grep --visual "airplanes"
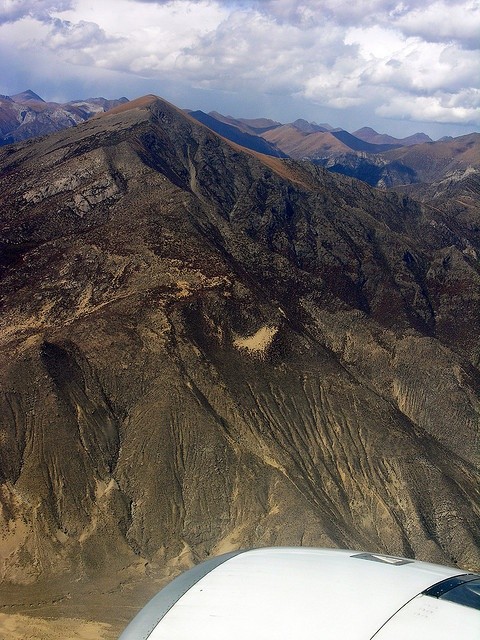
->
[120,545,480,640]
